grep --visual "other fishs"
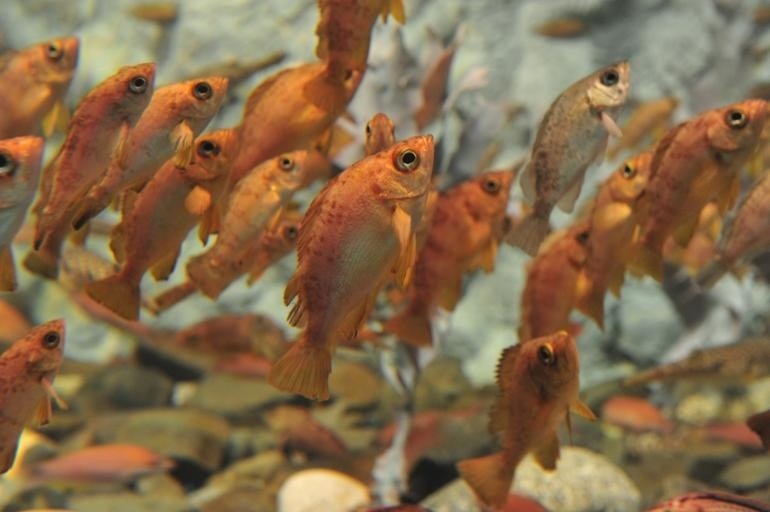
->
[0,0,769,512]
